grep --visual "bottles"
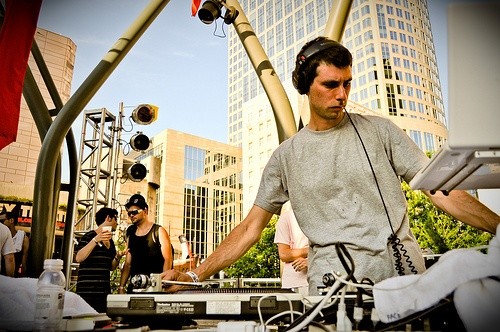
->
[32,258,66,332]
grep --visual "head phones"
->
[291,39,341,90]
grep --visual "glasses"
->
[0,218,11,224]
[127,209,144,217]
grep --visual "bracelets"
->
[92,238,102,247]
[186,271,199,282]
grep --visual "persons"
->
[161,36,500,332]
[75,207,130,314]
[3,211,29,278]
[118,194,174,294]
[0,222,15,277]
[273,200,309,293]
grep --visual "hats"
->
[123,194,149,210]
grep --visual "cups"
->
[102,226,112,240]
